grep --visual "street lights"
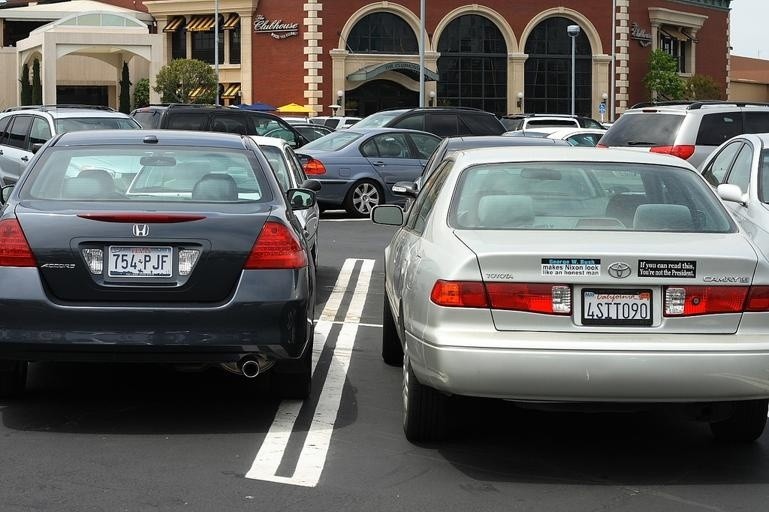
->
[566,24,581,114]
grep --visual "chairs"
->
[278,172,285,180]
[268,159,282,171]
[633,203,697,232]
[363,144,384,157]
[75,169,115,196]
[174,161,211,191]
[604,189,648,220]
[42,126,50,139]
[213,123,226,131]
[378,143,402,156]
[230,125,246,133]
[62,178,102,199]
[477,193,535,231]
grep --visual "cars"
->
[369,140,769,446]
[254,102,616,148]
[0,127,318,402]
[291,126,444,220]
[697,132,768,263]
[0,101,186,211]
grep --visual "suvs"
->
[128,101,313,150]
[592,99,769,194]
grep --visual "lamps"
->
[337,90,343,106]
[601,93,608,105]
[517,92,523,109]
[429,91,435,107]
